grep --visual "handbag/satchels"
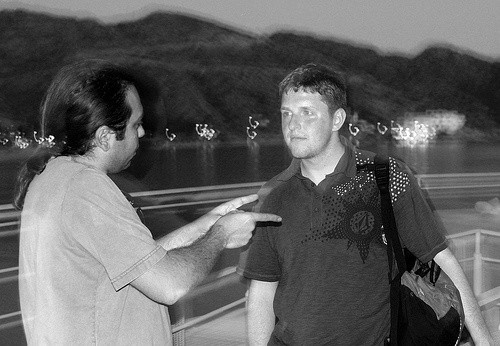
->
[374,152,465,346]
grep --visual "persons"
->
[236,63,495,346]
[11,61,284,346]
